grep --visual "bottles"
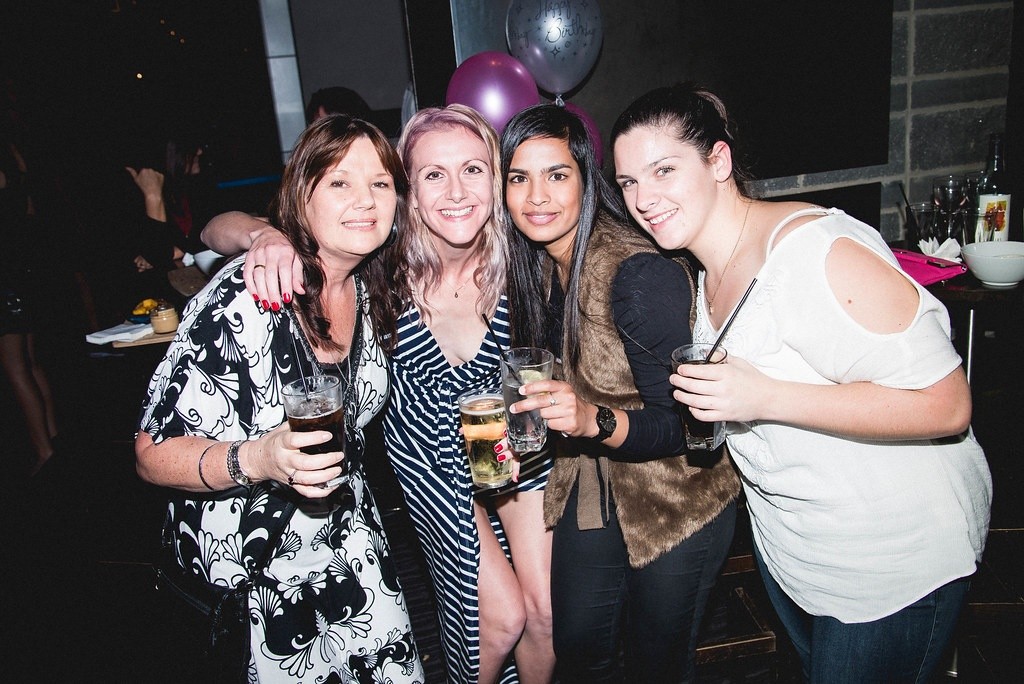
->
[974,131,1012,243]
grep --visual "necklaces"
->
[331,352,355,400]
[443,278,470,298]
[705,199,752,315]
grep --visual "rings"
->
[288,470,297,484]
[254,265,265,269]
[550,398,556,405]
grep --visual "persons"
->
[498,105,742,684]
[306,87,370,124]
[125,114,202,294]
[609,85,993,684]
[136,115,426,684]
[200,102,554,684]
[0,139,60,476]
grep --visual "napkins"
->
[917,237,962,263]
[86,322,154,345]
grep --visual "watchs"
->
[592,405,617,442]
[232,439,255,486]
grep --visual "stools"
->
[695,528,1024,684]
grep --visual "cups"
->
[905,204,938,254]
[500,347,553,452]
[457,387,513,487]
[962,212,997,245]
[282,375,348,487]
[670,343,727,451]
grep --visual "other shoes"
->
[24,453,65,481]
[51,434,64,448]
[90,344,128,358]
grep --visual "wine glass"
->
[933,175,968,239]
[963,175,980,228]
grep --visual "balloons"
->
[565,102,603,170]
[446,51,539,135]
[506,0,603,95]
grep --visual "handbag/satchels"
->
[137,565,251,684]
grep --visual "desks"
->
[111,320,174,347]
[888,240,1024,385]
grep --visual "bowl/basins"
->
[960,240,1024,287]
[193,250,226,277]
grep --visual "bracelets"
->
[199,444,215,490]
[227,440,241,481]
